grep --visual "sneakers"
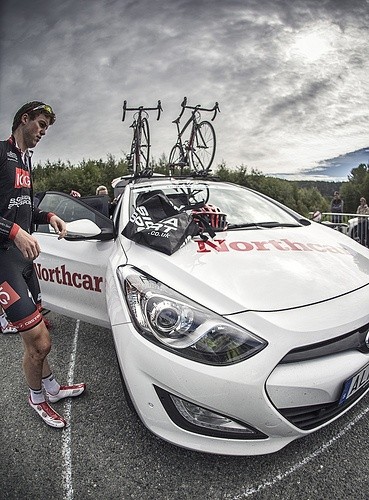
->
[1,323,19,333]
[28,395,66,428]
[46,383,86,403]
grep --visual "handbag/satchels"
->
[119,194,215,256]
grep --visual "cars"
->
[33,173,369,457]
[346,216,369,242]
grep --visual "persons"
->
[0,100,85,428]
[68,185,119,218]
[331,191,343,223]
[357,197,369,247]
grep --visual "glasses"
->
[26,105,52,114]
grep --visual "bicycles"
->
[120,97,164,176]
[169,97,220,174]
